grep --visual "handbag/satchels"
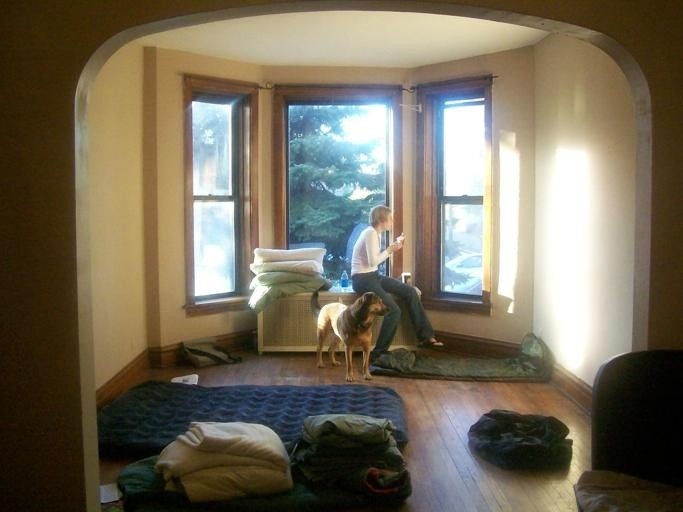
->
[180,341,233,368]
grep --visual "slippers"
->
[417,340,447,350]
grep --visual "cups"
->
[397,237,405,245]
[400,272,410,285]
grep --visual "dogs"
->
[310,290,392,382]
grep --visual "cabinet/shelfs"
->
[257,289,421,354]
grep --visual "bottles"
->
[339,270,348,290]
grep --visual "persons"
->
[350,205,447,363]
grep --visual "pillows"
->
[248,247,326,309]
